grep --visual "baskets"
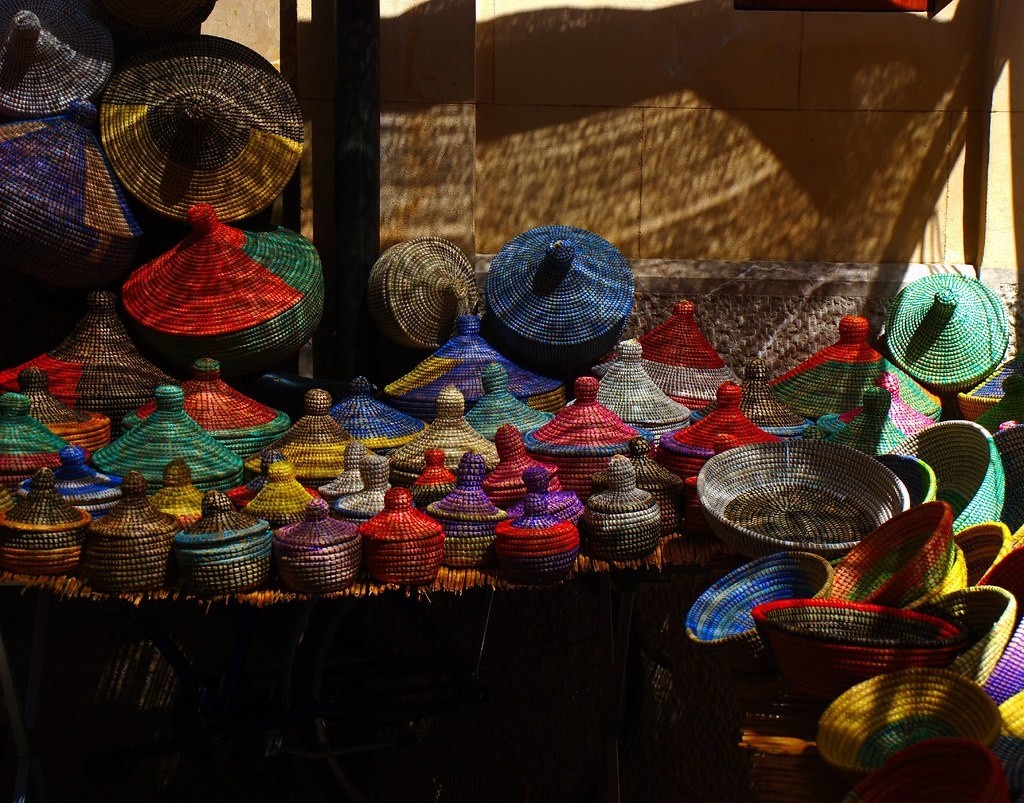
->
[681,418,1024,803]
